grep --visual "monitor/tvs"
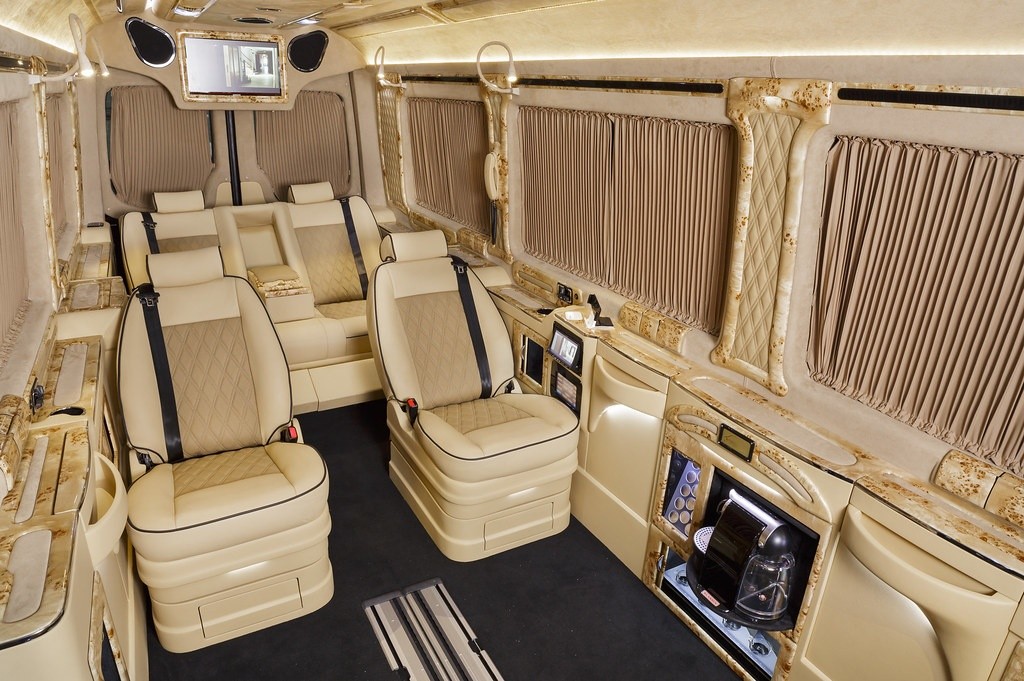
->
[555,372,577,408]
[548,328,581,369]
[101,621,121,681]
[176,29,289,105]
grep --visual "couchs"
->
[116,246,335,653]
[366,230,581,563]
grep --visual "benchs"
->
[118,180,385,416]
